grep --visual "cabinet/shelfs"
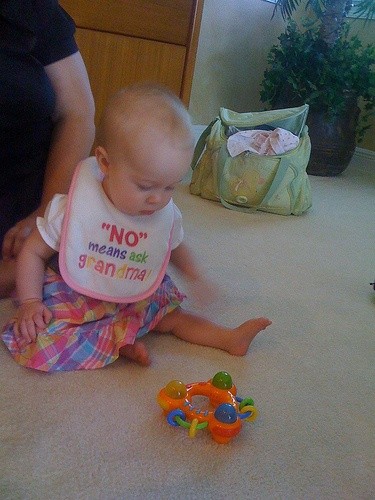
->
[58,0,205,158]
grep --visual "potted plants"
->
[257,0,375,177]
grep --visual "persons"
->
[0,85,273,372]
[0,0,96,298]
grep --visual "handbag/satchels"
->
[189,104,313,216]
